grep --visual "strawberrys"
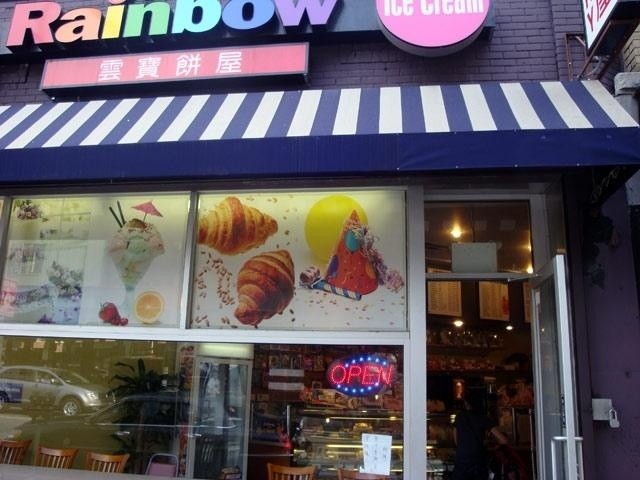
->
[98,302,119,322]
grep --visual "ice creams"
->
[104,200,165,292]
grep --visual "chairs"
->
[338,468,391,479]
[142,453,180,475]
[267,462,317,478]
[1,439,30,466]
[85,451,130,472]
[32,445,78,468]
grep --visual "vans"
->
[1,364,118,418]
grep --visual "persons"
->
[453,388,529,480]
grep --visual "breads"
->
[196,196,278,255]
[234,250,295,326]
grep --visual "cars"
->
[11,391,244,479]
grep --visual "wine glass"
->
[108,251,156,323]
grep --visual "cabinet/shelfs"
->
[291,407,446,480]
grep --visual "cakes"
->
[300,264,319,284]
[0,200,60,322]
[323,210,379,295]
